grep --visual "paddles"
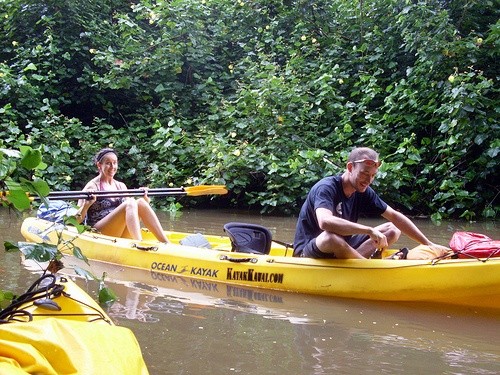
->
[0,185,228,203]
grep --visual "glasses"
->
[350,159,383,168]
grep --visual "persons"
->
[292,147,435,259]
[75,148,169,243]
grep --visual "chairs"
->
[223,222,295,255]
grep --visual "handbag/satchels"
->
[37,199,78,224]
[450,231,500,259]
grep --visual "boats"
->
[0,265,150,374]
[21,217,500,310]
